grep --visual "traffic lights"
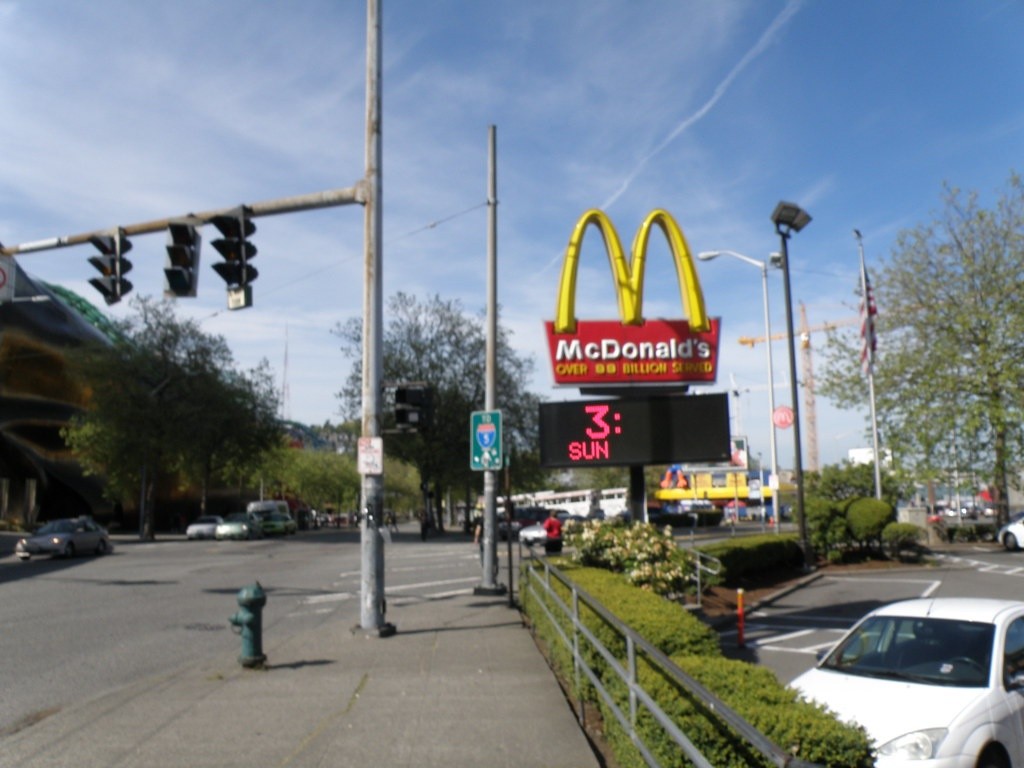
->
[209,203,259,288]
[87,235,133,303]
[165,222,202,298]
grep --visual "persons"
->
[474,514,498,581]
[386,512,398,535]
[421,512,428,541]
[544,512,562,555]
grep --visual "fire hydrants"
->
[228,579,267,669]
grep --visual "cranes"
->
[738,298,858,475]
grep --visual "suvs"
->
[214,511,263,541]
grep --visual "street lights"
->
[697,249,779,535]
[769,199,816,574]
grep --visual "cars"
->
[997,515,1024,550]
[260,511,298,537]
[783,596,1024,768]
[918,491,1003,544]
[296,507,395,531]
[664,499,724,528]
[467,504,587,547]
[14,517,112,561]
[186,515,223,541]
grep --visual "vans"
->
[244,499,291,524]
